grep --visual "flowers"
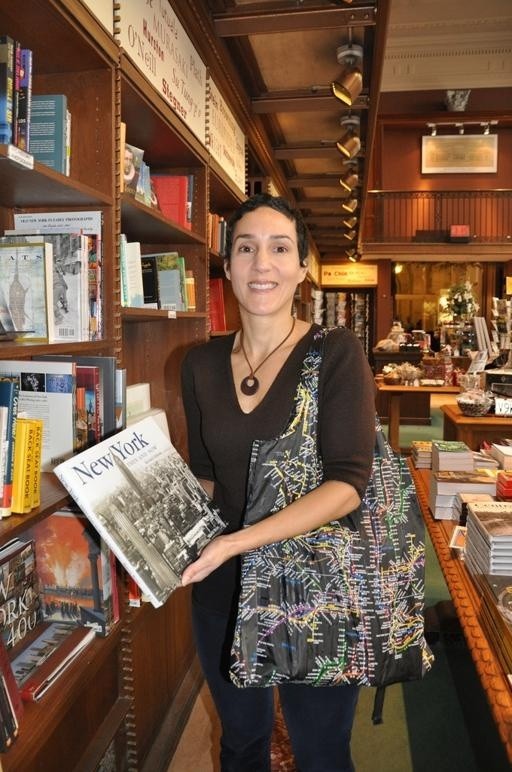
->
[443,280,480,315]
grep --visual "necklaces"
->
[237,313,297,398]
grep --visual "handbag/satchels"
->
[223,325,438,690]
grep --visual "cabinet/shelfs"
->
[0,1,250,772]
[491,295,512,370]
[290,260,378,376]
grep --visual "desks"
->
[406,455,512,772]
[373,374,463,451]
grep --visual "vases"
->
[451,315,463,324]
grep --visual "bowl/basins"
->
[455,397,491,417]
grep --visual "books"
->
[3,356,126,473]
[121,121,228,331]
[0,653,25,747]
[1,209,104,345]
[0,38,72,177]
[20,505,142,638]
[126,383,169,439]
[410,438,512,685]
[2,539,43,648]
[3,380,42,518]
[5,622,96,701]
[53,414,224,609]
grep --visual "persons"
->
[182,193,375,771]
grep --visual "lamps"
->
[341,192,358,241]
[344,247,359,263]
[338,158,359,192]
[336,115,362,159]
[331,45,365,106]
